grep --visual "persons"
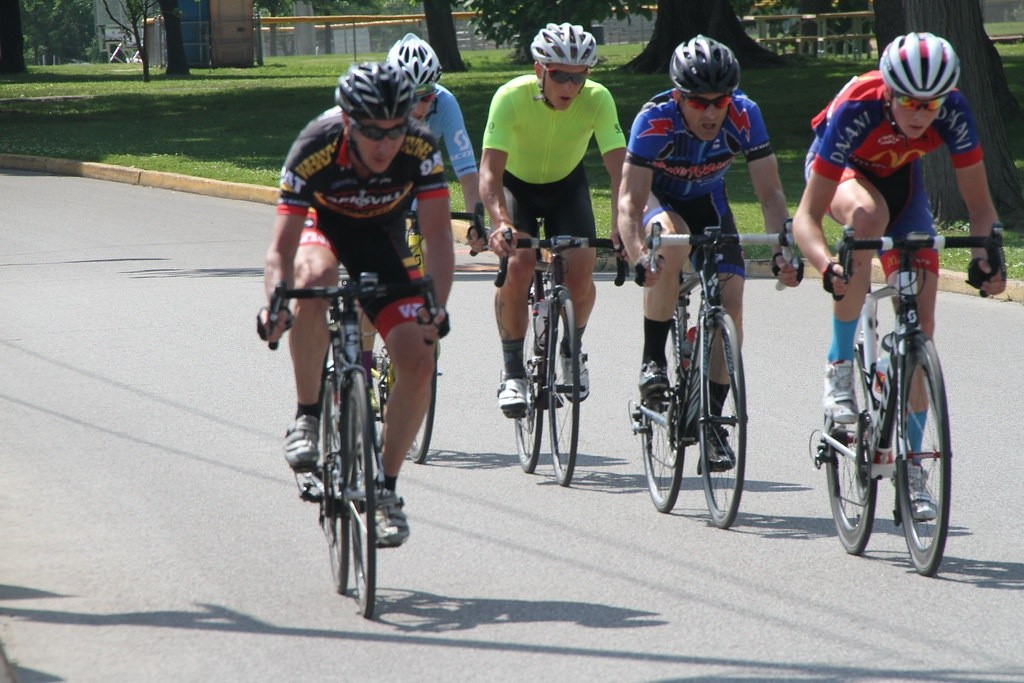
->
[356,32,491,414]
[477,23,632,410]
[792,32,1006,520]
[617,33,804,471]
[258,60,455,544]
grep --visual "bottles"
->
[680,326,695,368]
[536,297,550,338]
[872,334,895,401]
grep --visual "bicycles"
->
[494,222,631,488]
[808,223,1007,576]
[628,217,804,529]
[264,278,434,620]
[363,201,487,467]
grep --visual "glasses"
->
[542,64,591,85]
[892,88,948,110]
[349,116,409,141]
[681,91,733,110]
[413,88,440,105]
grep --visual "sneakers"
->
[640,360,669,393]
[697,431,736,473]
[559,342,589,403]
[821,346,859,423]
[282,414,320,466]
[907,461,937,520]
[497,368,528,411]
[373,470,410,545]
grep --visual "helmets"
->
[879,32,961,97]
[669,34,741,94]
[382,33,442,95]
[335,61,416,120]
[530,22,598,67]
